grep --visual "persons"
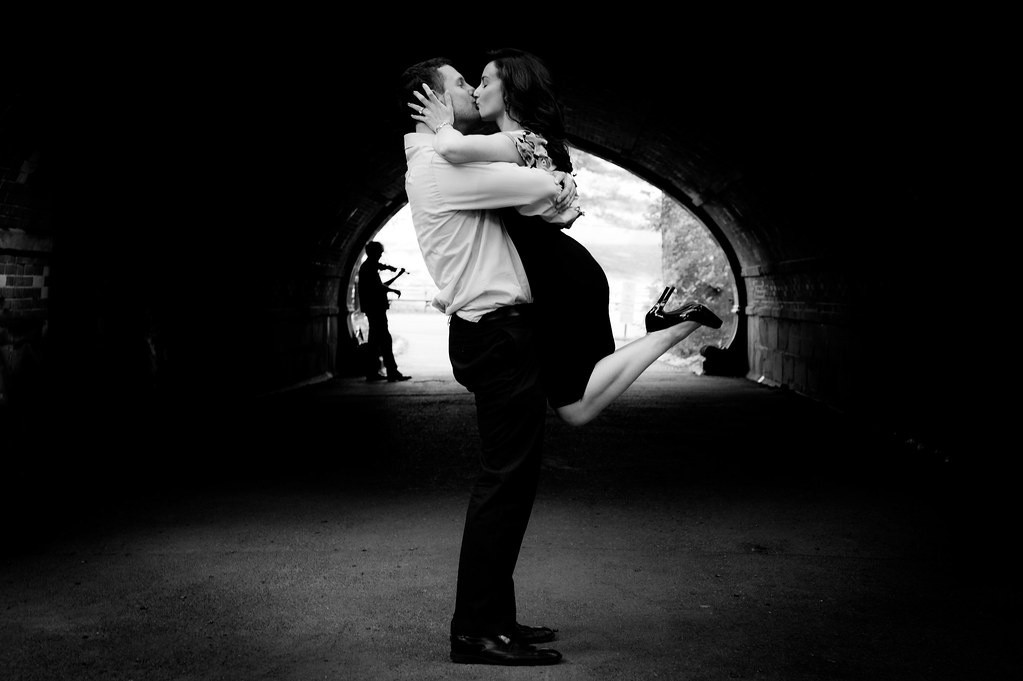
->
[358,241,412,384]
[408,47,723,427]
[403,56,584,667]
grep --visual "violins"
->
[378,262,410,274]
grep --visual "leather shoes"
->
[451,634,563,665]
[502,621,555,644]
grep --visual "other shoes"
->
[387,370,412,382]
[366,375,386,382]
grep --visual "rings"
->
[419,107,425,115]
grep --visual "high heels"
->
[645,285,724,331]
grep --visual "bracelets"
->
[435,121,454,134]
